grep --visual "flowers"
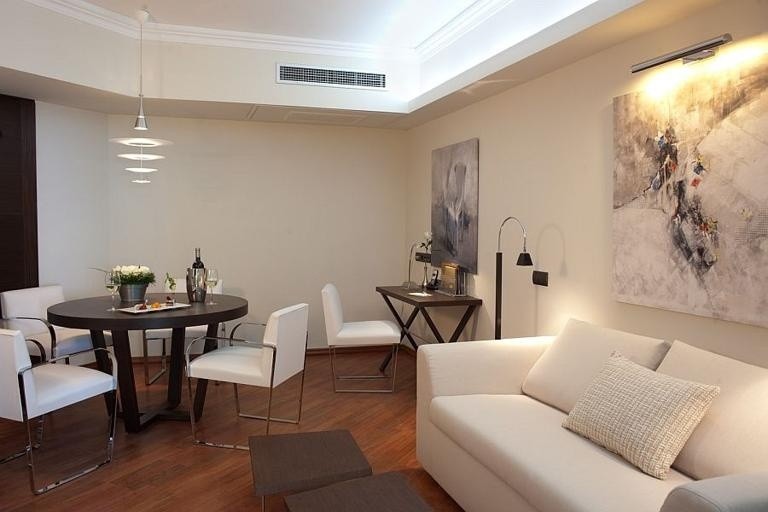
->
[101,263,157,285]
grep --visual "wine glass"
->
[105,272,122,311]
[204,268,219,305]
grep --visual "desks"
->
[372,284,483,376]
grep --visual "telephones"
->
[426,269,442,291]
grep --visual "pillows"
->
[562,352,720,478]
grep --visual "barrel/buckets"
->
[115,283,147,302]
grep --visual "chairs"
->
[136,277,229,384]
[2,326,121,496]
[1,282,117,379]
[180,302,314,452]
[321,284,400,394]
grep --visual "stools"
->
[238,424,372,512]
[277,466,432,512]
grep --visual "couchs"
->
[406,321,767,511]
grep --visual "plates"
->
[116,302,191,314]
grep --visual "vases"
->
[112,282,142,307]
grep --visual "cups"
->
[163,278,176,305]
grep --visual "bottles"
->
[186,247,207,302]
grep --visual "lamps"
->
[493,214,534,342]
[131,4,149,132]
[624,31,733,76]
